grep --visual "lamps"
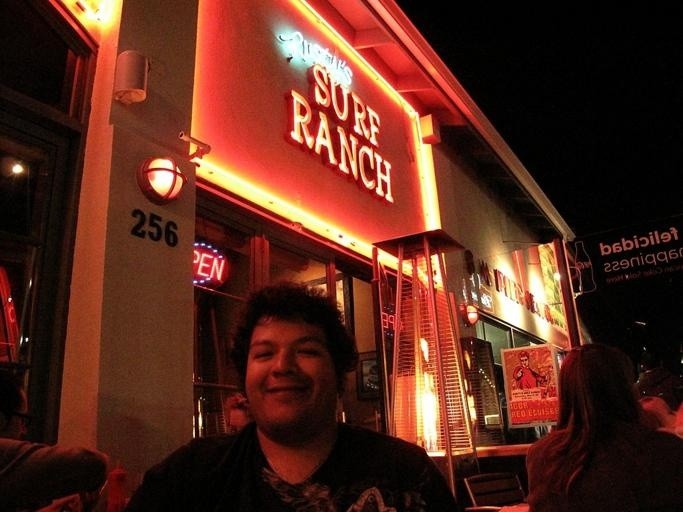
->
[114,51,151,104]
[460,303,480,327]
[137,155,189,206]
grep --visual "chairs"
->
[464,468,527,512]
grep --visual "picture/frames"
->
[355,349,381,402]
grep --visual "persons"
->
[524,343,683,512]
[124,282,458,512]
[513,351,548,390]
[0,369,105,512]
[224,392,255,434]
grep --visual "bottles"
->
[573,239,596,292]
[106,458,128,511]
